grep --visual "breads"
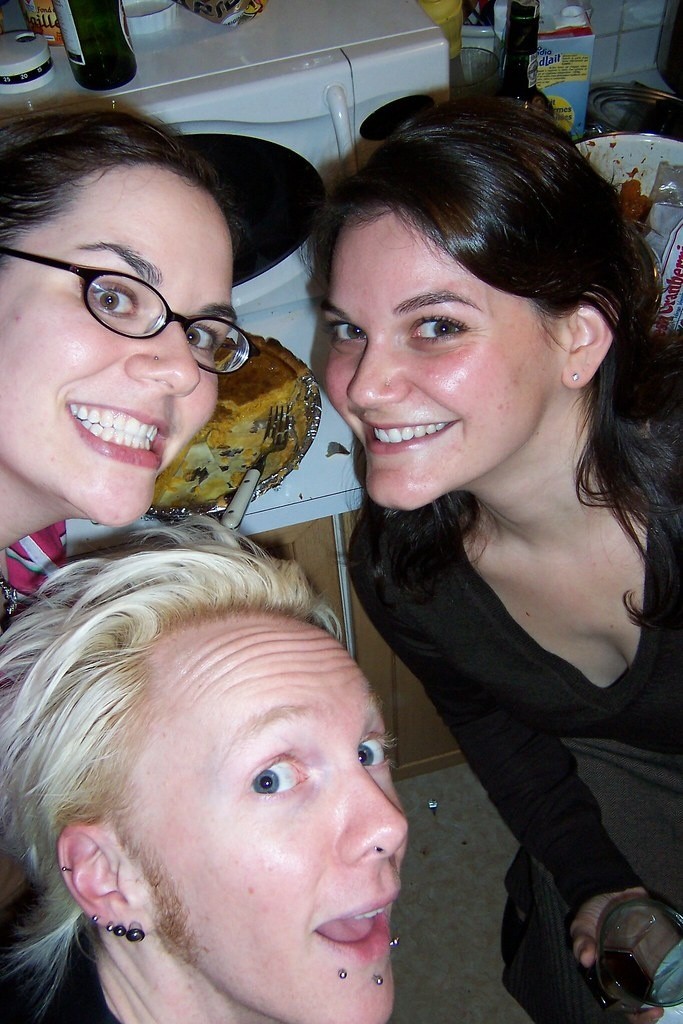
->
[148,332,322,520]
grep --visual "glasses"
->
[0,243,261,375]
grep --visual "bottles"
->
[504,0,540,105]
[51,0,137,91]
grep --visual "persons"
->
[319,98,683,1024]
[0,521,410,1023]
[0,104,324,639]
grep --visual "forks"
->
[217,405,290,532]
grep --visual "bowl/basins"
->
[122,0,177,35]
[575,133,683,196]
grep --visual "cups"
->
[449,46,501,103]
[596,896,683,1012]
[417,0,464,60]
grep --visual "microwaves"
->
[0,0,451,569]
